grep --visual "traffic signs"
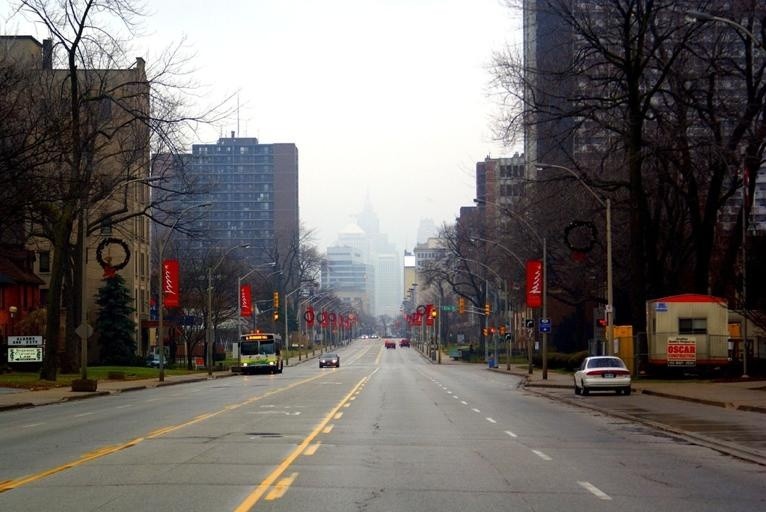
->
[442,305,456,311]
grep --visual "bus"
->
[237,331,283,375]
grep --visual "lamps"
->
[8,306,17,318]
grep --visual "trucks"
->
[147,344,169,368]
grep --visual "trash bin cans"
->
[488,358,495,368]
[431,351,434,359]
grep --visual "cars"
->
[360,334,410,350]
[316,352,339,368]
[572,355,633,397]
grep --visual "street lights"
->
[451,195,550,382]
[530,158,614,358]
[680,5,766,59]
[284,281,358,365]
[235,260,277,339]
[252,268,284,331]
[69,174,160,393]
[397,282,440,364]
[157,199,215,383]
[205,242,253,378]
